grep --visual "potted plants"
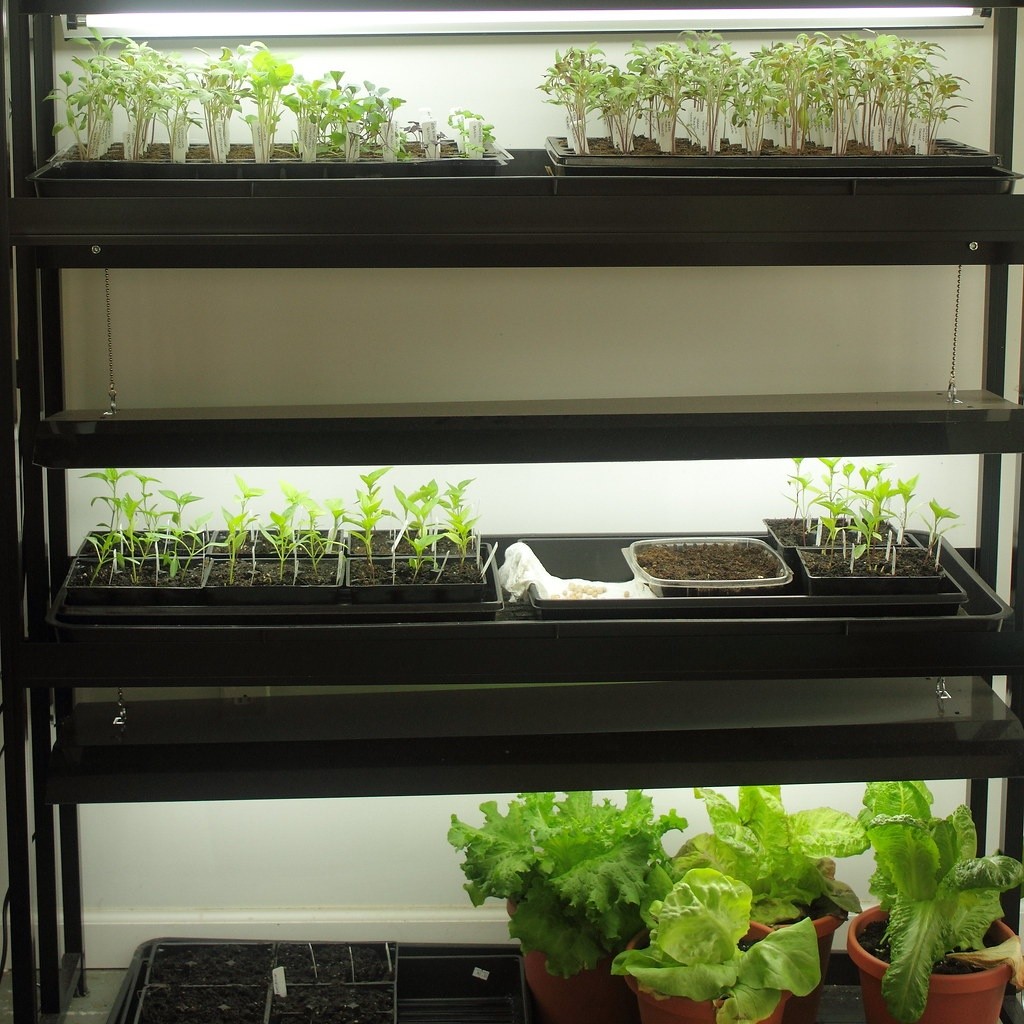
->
[43,25,1023,1024]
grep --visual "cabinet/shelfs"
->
[0,0,1022,1023]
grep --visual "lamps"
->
[35,677,1022,805]
[48,259,1024,469]
